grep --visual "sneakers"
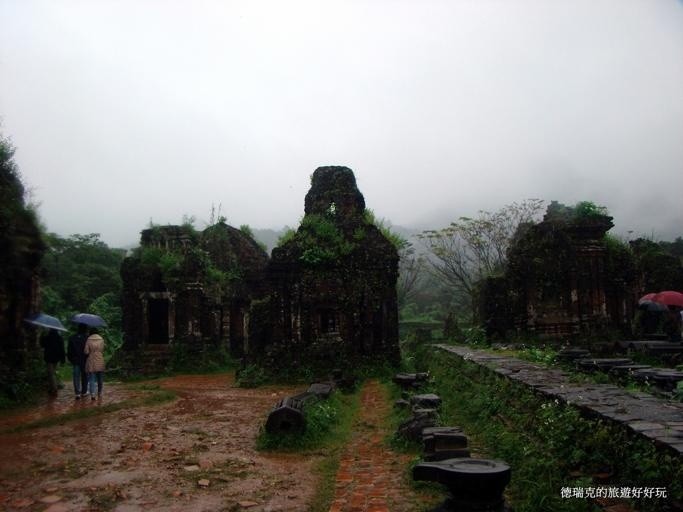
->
[76,390,102,400]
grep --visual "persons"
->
[38,327,65,396]
[66,323,90,399]
[82,327,105,399]
[637,306,682,344]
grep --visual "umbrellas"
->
[69,312,108,332]
[638,292,667,307]
[650,290,682,308]
[21,312,68,333]
